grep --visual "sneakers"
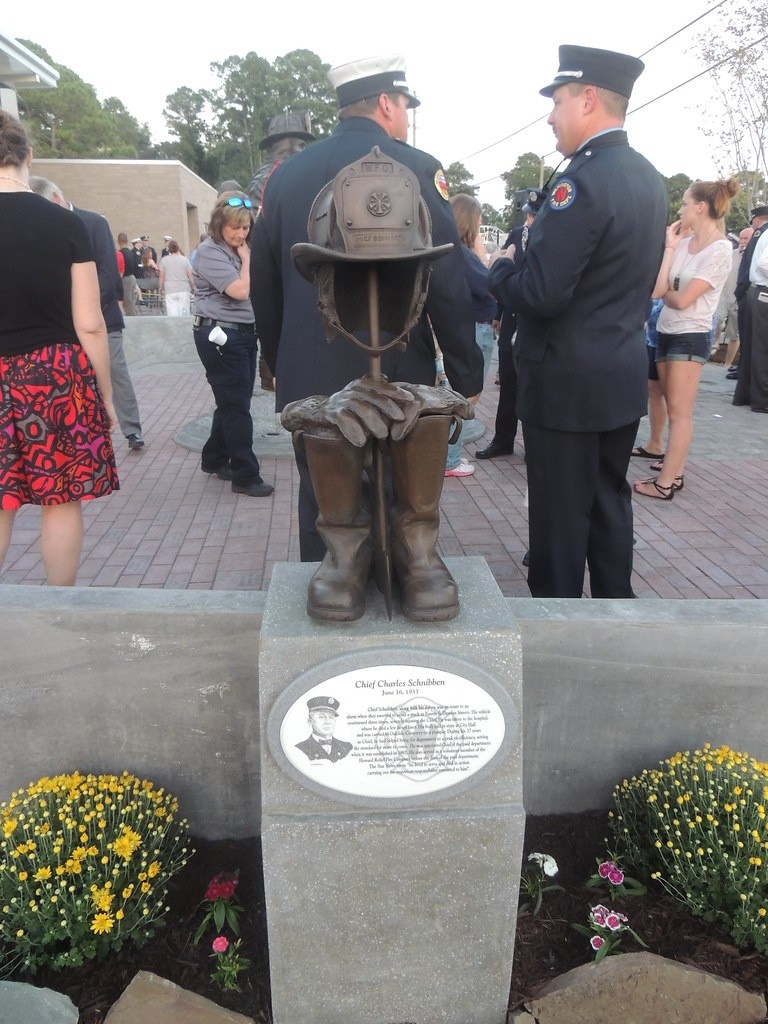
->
[232,482,274,497]
[201,462,232,480]
[445,464,474,476]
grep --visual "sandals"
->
[647,474,683,490]
[634,480,674,500]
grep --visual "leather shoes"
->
[726,370,738,379]
[475,442,513,459]
[728,366,739,372]
[128,434,145,450]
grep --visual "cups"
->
[208,325,227,345]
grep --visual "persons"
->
[294,696,354,765]
[0,44,768,601]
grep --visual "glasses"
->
[223,197,253,209]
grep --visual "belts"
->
[194,317,257,335]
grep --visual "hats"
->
[522,187,546,215]
[539,45,645,98]
[130,235,172,244]
[749,206,768,224]
[327,53,421,109]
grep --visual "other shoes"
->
[262,379,275,391]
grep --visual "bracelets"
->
[665,243,677,250]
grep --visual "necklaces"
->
[0,177,32,190]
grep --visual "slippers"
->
[650,458,664,471]
[631,446,665,458]
